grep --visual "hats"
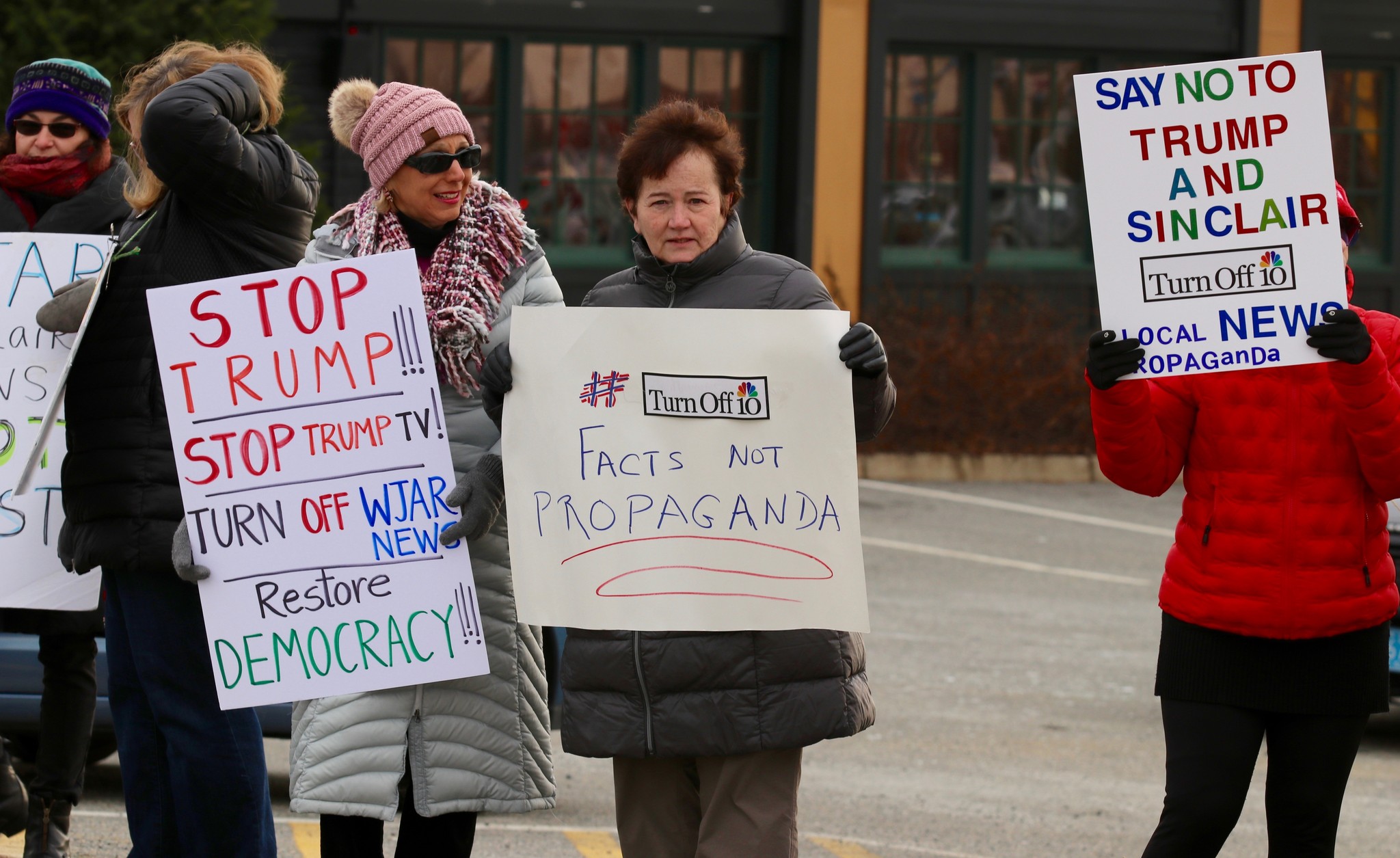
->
[328,77,476,192]
[5,58,109,144]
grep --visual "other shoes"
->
[22,785,72,858]
[0,735,31,836]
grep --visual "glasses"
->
[10,117,81,138]
[130,142,146,161]
[402,144,482,174]
[1339,217,1363,247]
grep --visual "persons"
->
[1083,180,1400,858]
[0,58,134,858]
[476,103,899,858]
[56,41,322,858]
[175,78,566,858]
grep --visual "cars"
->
[878,177,1085,254]
[510,172,634,248]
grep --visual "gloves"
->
[838,324,888,387]
[36,276,97,334]
[1084,332,1146,390]
[477,343,513,400]
[57,518,100,575]
[1306,310,1373,363]
[172,518,211,581]
[440,453,504,545]
